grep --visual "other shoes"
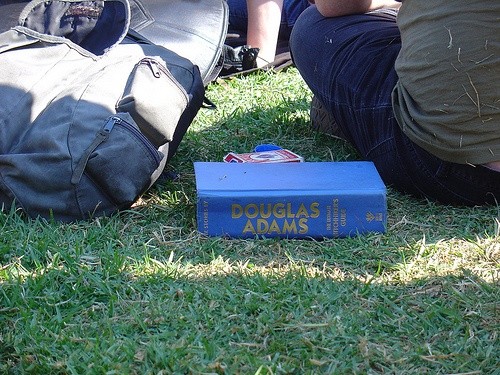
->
[311,94,349,142]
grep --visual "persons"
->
[289,0,500,210]
[216,0,318,78]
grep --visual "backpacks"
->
[1,0,230,223]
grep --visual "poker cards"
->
[223,149,304,163]
